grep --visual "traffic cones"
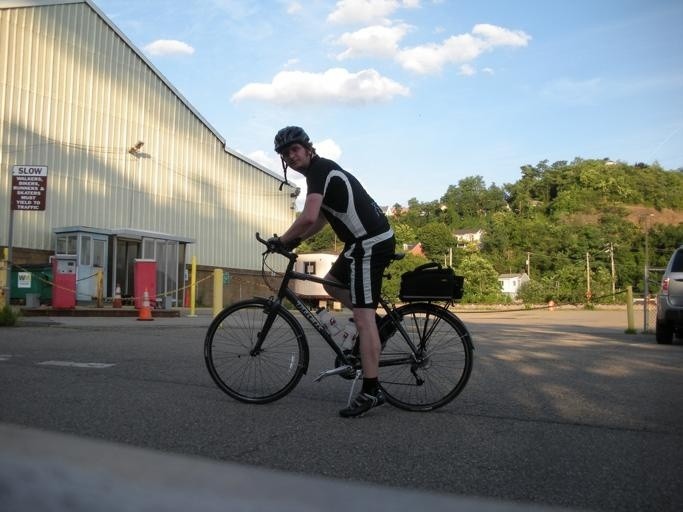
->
[112,283,122,308]
[137,287,154,320]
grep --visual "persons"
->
[265,125,396,418]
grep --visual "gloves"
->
[269,237,302,251]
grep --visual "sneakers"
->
[339,390,386,418]
[375,313,381,323]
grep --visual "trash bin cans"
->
[26,293,41,307]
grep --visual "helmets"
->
[274,126,311,150]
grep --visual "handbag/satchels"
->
[400,263,465,299]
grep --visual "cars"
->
[656,245,683,344]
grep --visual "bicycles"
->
[204,232,475,412]
